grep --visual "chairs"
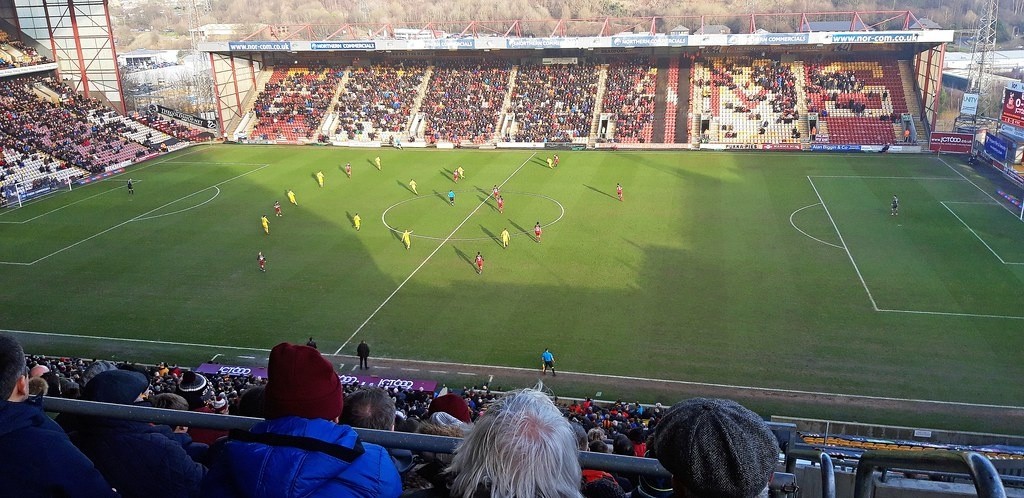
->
[798,432,1024,468]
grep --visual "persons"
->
[249,50,915,151]
[474,252,484,275]
[501,228,510,248]
[969,155,974,165]
[493,185,504,214]
[316,170,324,187]
[352,213,361,231]
[375,156,381,170]
[0,35,202,203]
[891,195,898,217]
[288,189,298,206]
[260,215,270,235]
[616,183,622,201]
[448,190,455,206]
[256,252,267,271]
[409,179,418,196]
[546,154,559,169]
[401,229,413,249]
[274,201,282,217]
[453,166,465,183]
[345,163,351,178]
[0,333,779,498]
[531,222,543,242]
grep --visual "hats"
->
[82,369,149,405]
[30,363,50,379]
[265,342,344,420]
[81,360,119,387]
[654,396,780,498]
[152,392,191,412]
[174,370,211,407]
[429,393,470,423]
[213,396,227,411]
